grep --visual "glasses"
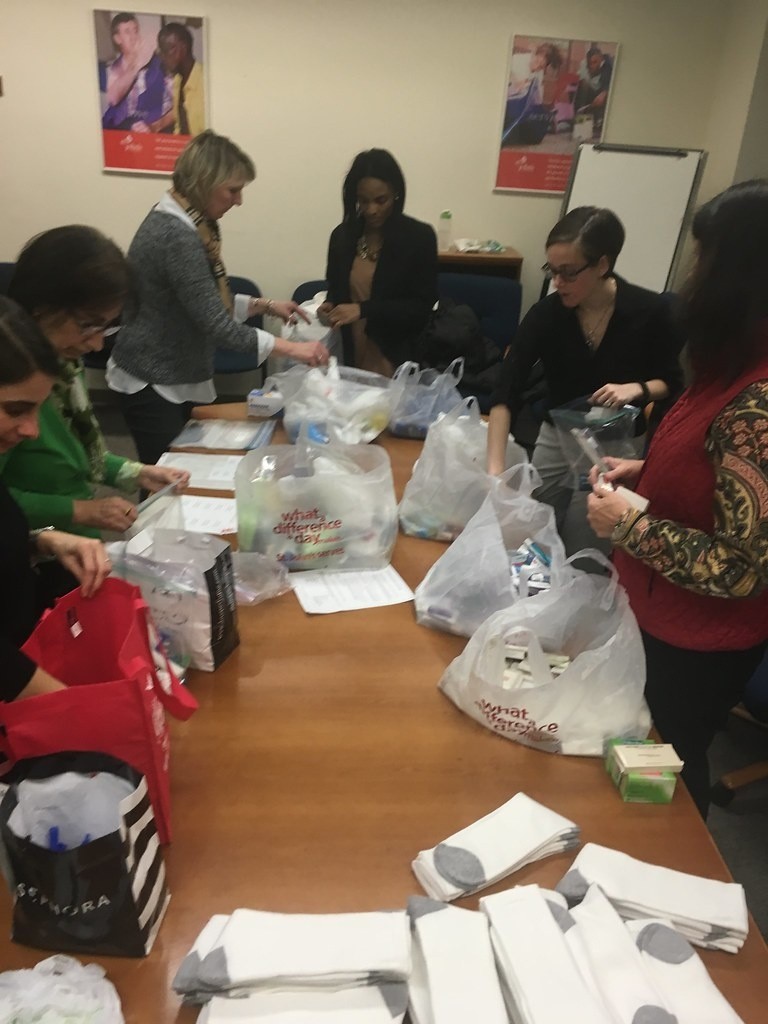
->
[71,310,127,336]
[540,261,597,283]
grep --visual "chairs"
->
[1,274,331,390]
[430,271,520,402]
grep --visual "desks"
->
[1,395,768,1024]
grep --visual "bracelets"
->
[253,298,262,310]
[639,380,651,402]
[614,508,632,529]
[264,299,274,313]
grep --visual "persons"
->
[498,42,563,149]
[316,145,439,385]
[8,223,189,541]
[583,176,768,827]
[98,13,175,134]
[0,292,111,708]
[158,22,206,137]
[485,205,688,573]
[107,130,330,507]
[577,47,614,144]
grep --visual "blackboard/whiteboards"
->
[538,136,709,302]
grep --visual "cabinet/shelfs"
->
[431,247,524,312]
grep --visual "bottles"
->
[436,209,454,253]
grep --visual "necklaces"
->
[582,295,615,349]
[357,233,382,262]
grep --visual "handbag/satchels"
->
[0,525,241,957]
[389,356,654,756]
[233,355,407,569]
[276,290,342,372]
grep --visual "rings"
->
[126,507,132,517]
[318,354,322,362]
[103,557,111,563]
[287,314,293,321]
